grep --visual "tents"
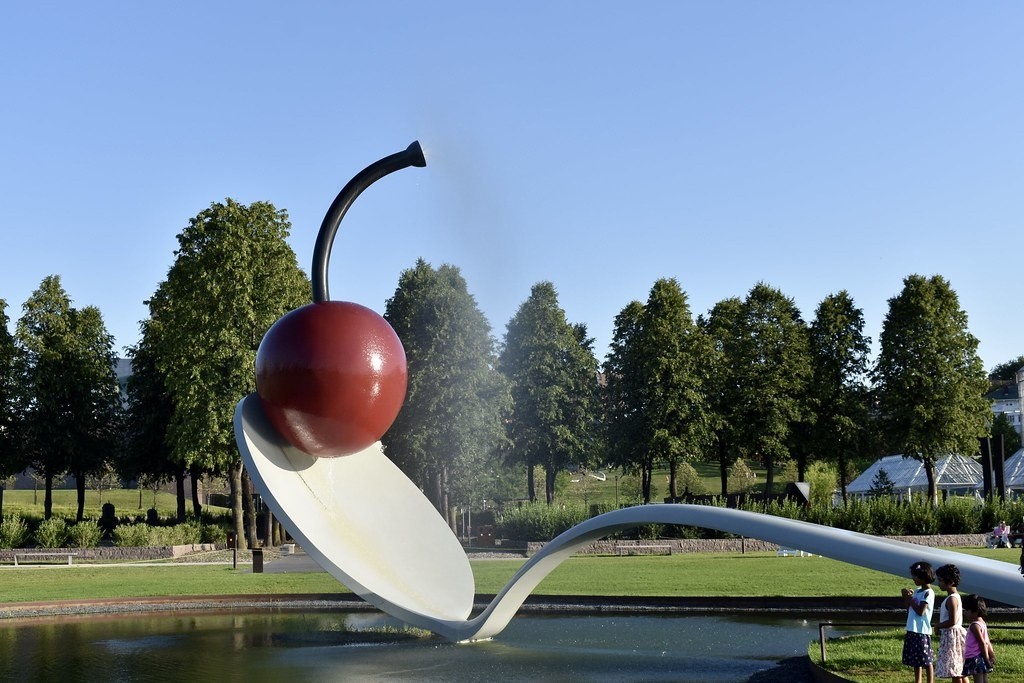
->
[845,448,1024,506]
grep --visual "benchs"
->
[616,546,672,556]
[14,553,78,565]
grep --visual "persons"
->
[992,521,1008,549]
[930,564,969,683]
[901,561,936,683]
[961,592,996,683]
